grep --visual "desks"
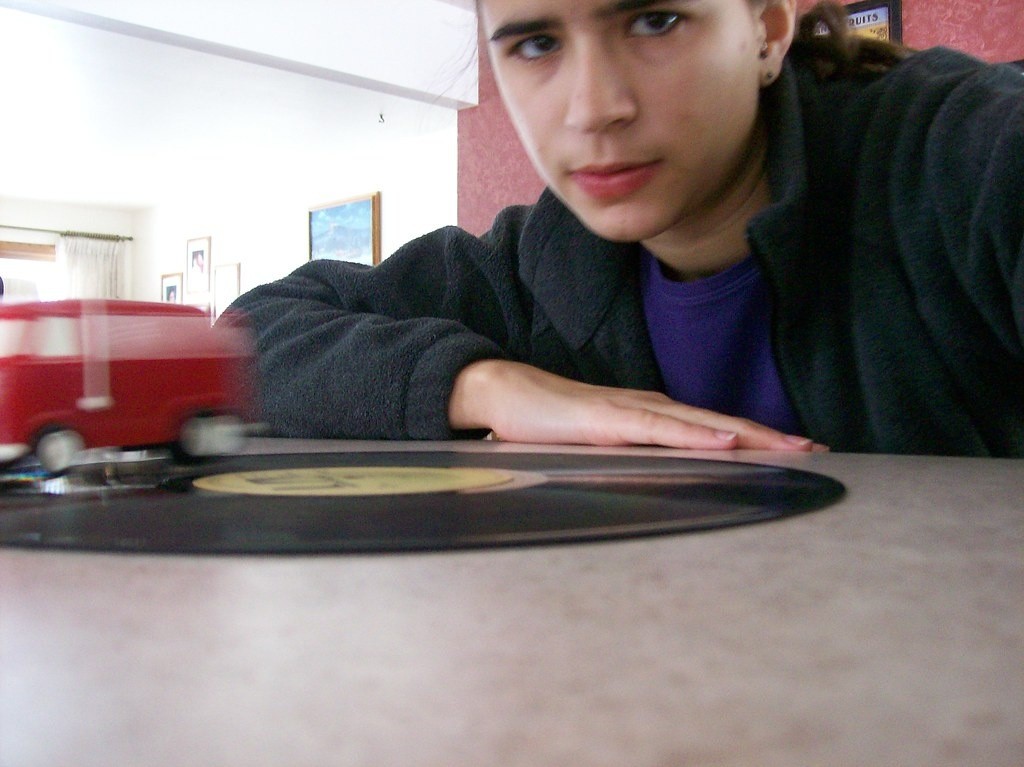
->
[0,434,1024,767]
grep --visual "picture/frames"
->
[214,262,240,321]
[810,0,902,47]
[306,191,382,267]
[161,272,182,304]
[186,236,211,308]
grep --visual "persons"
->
[210,1,1023,451]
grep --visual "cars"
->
[0,298,256,470]
